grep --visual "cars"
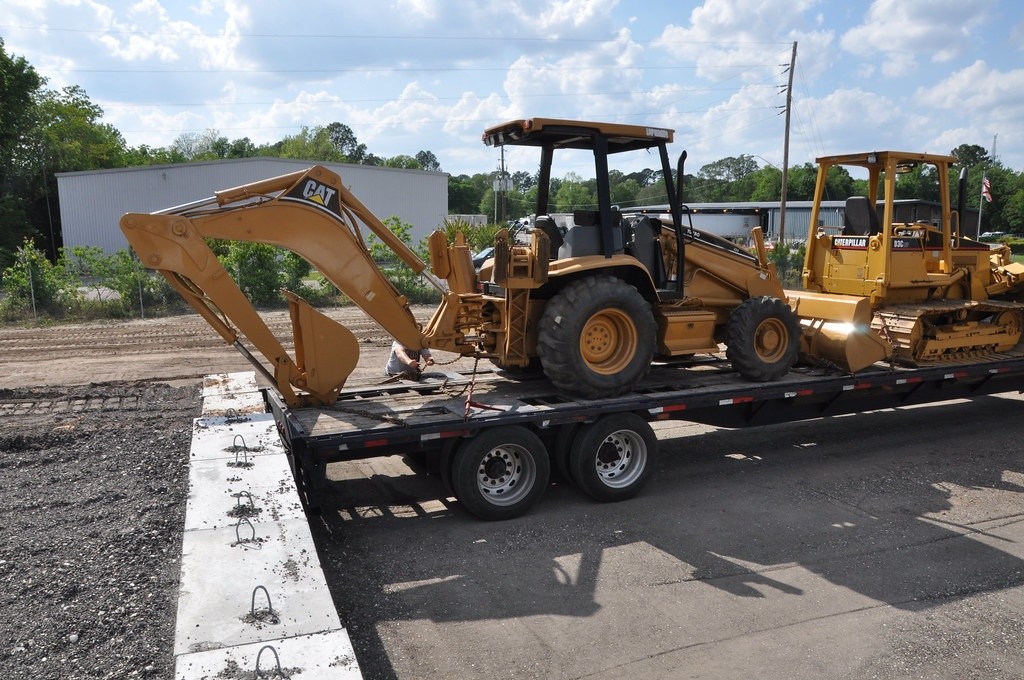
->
[472,245,495,268]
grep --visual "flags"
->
[984,175,991,187]
[982,185,992,203]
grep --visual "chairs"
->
[536,215,565,260]
[841,196,878,236]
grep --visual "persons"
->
[383,341,434,375]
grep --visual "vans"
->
[981,231,1007,237]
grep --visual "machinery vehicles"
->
[118,117,804,397]
[783,150,1024,376]
